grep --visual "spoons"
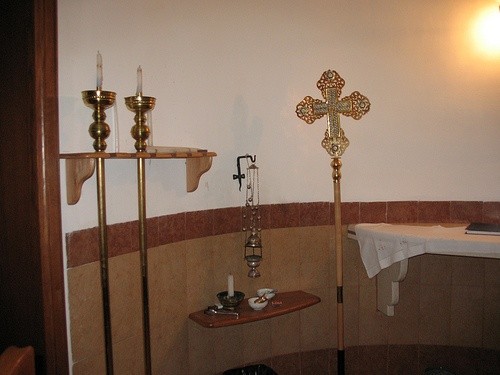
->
[204,307,239,316]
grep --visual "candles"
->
[137,65,142,92]
[96,51,103,86]
[228,273,234,296]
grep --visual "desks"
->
[347,222,500,317]
[188,289,321,329]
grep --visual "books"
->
[465,222,500,236]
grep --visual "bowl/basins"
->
[248,297,268,311]
[217,290,245,310]
[257,288,276,300]
